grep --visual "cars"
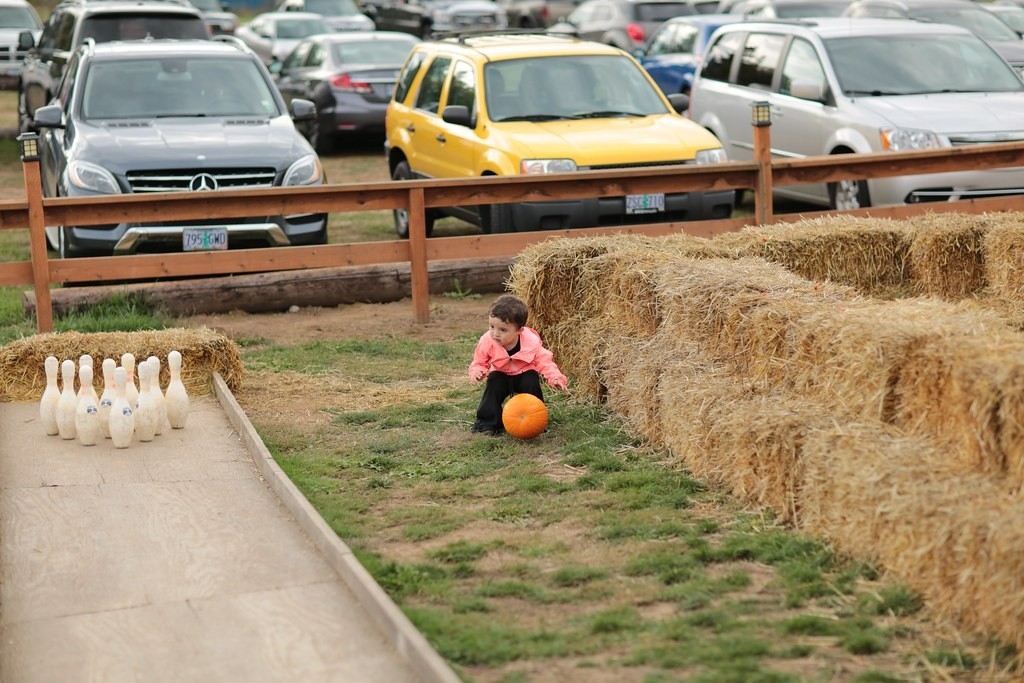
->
[633,15,750,106]
[274,0,376,35]
[0,0,46,76]
[736,1,844,17]
[234,12,324,72]
[273,32,425,153]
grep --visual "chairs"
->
[489,68,523,117]
[180,66,244,112]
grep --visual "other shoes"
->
[481,429,494,436]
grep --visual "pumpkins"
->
[502,393,547,439]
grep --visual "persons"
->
[467,294,572,437]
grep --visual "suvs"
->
[34,34,327,285]
[383,27,736,238]
[21,0,210,122]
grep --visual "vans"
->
[688,17,1023,212]
